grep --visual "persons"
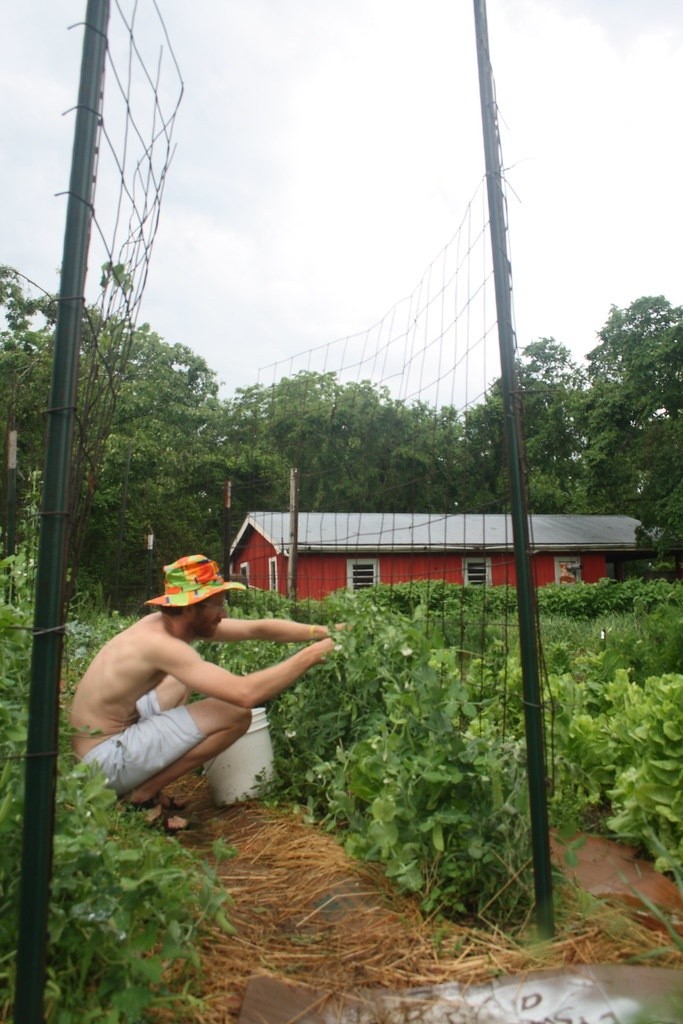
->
[68,555,352,835]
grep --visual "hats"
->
[144,554,246,607]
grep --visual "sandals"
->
[127,797,192,834]
[157,792,186,811]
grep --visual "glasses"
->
[202,600,227,611]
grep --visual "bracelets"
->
[310,625,317,638]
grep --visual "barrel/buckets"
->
[202,707,274,805]
